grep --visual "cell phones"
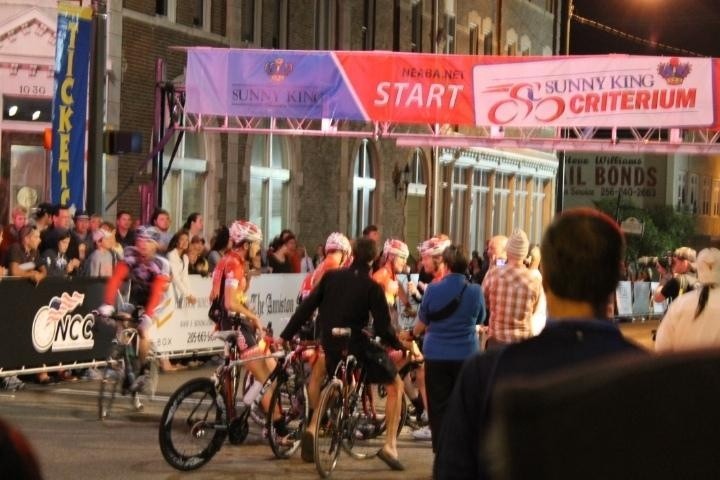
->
[496,258,506,266]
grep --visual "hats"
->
[695,248,720,285]
[72,210,90,220]
[92,228,111,243]
[673,247,698,270]
[506,228,529,257]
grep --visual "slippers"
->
[301,432,315,463]
[376,446,405,471]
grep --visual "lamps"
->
[397,162,412,198]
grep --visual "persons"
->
[429,207,720,478]
[208,219,546,470]
[1,203,325,393]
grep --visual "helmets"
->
[229,220,263,244]
[382,238,410,258]
[134,224,163,245]
[324,232,353,256]
[415,235,451,256]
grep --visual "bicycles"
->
[211,328,429,480]
[158,332,310,473]
[91,307,159,422]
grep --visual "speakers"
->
[104,131,140,153]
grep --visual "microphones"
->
[404,265,417,301]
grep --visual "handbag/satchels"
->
[426,295,465,320]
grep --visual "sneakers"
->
[1,360,204,391]
[412,424,432,442]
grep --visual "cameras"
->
[638,250,675,275]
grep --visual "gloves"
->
[98,304,116,317]
[137,315,152,332]
